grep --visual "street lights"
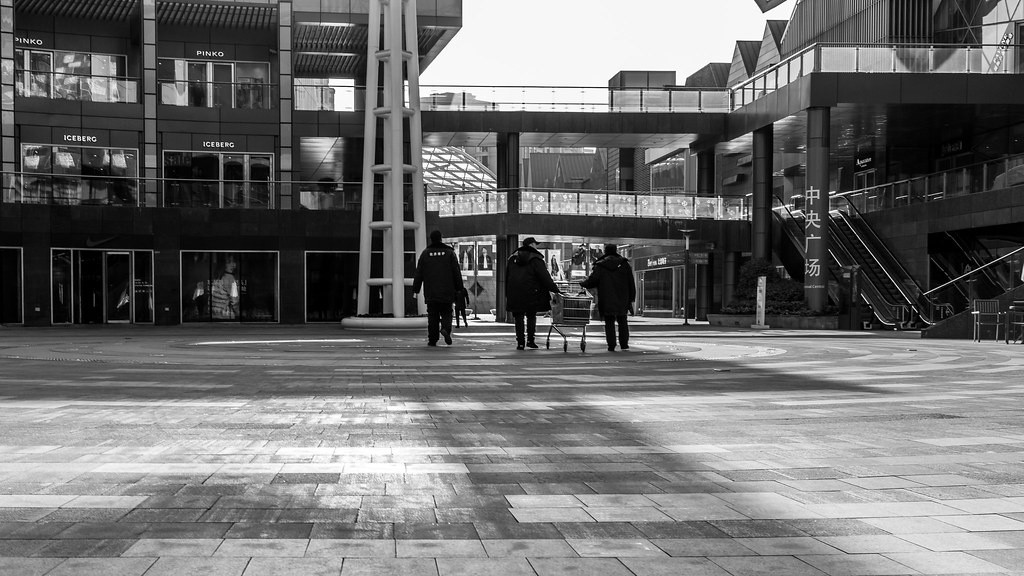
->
[679,229,695,325]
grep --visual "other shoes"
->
[441,329,452,345]
[517,344,524,349]
[455,326,460,328]
[428,341,436,346]
[465,323,468,327]
[527,340,539,348]
[608,346,615,351]
[620,344,629,349]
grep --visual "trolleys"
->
[546,281,592,352]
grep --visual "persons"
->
[477,246,493,270]
[591,245,603,262]
[503,236,564,351]
[572,243,586,264]
[193,253,241,320]
[459,244,474,270]
[455,288,470,328]
[411,231,465,346]
[550,254,559,276]
[579,245,636,352]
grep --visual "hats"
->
[430,230,442,239]
[523,237,542,246]
